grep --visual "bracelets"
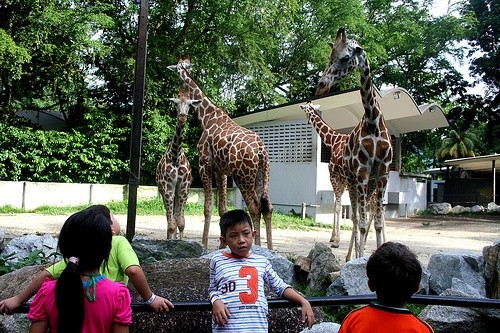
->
[210,296,220,305]
[143,292,156,305]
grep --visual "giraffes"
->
[155,85,192,240]
[300,27,394,264]
[166,55,274,250]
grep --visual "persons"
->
[337,241,434,333]
[0,205,174,316]
[207,209,315,333]
[26,212,132,333]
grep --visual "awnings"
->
[444,154,500,202]
